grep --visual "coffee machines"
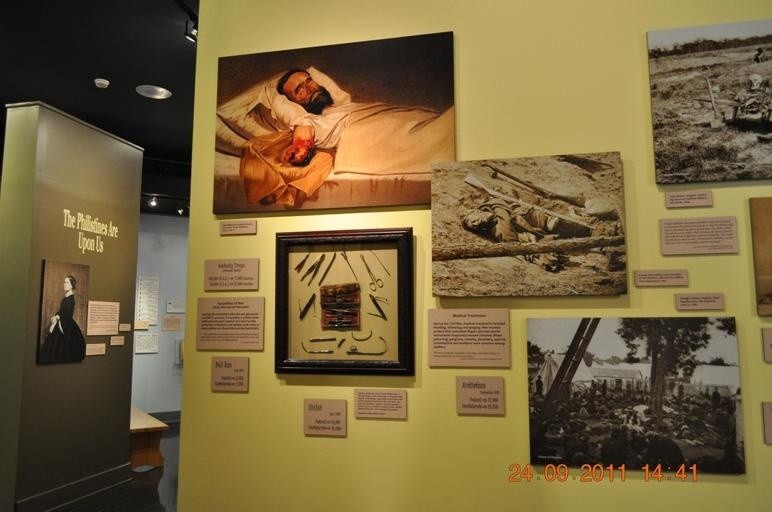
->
[360,254,384,291]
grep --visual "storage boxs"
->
[130,408,169,469]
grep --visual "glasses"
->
[183,16,197,45]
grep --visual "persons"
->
[276,68,455,170]
[37,276,85,363]
[464,200,592,257]
[529,373,745,475]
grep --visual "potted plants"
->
[275,226,415,377]
